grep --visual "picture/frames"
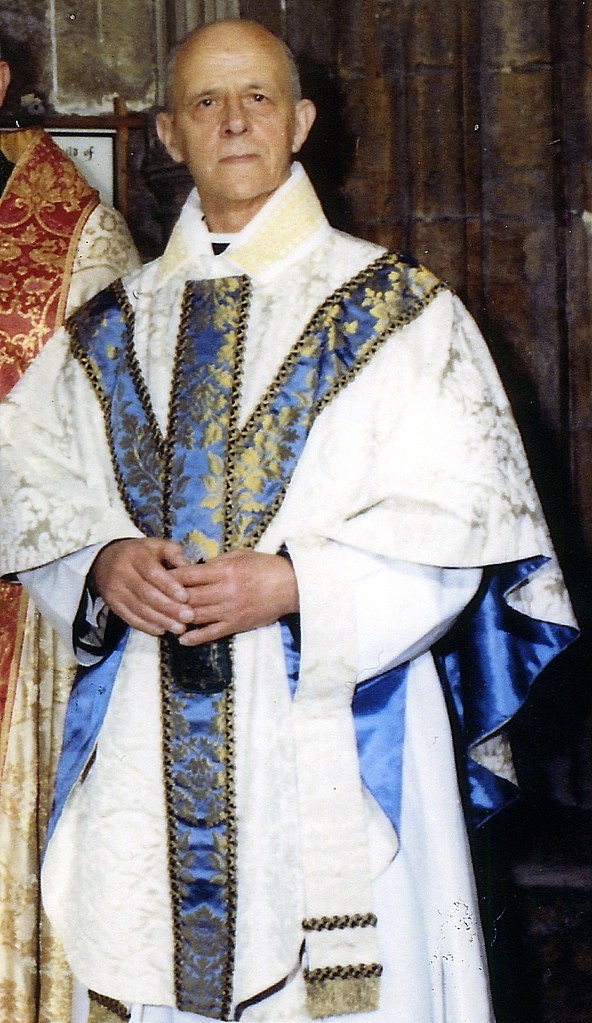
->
[43,97,145,216]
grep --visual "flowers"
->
[20,93,46,115]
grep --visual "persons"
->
[0,34,140,1022]
[0,18,583,1023]
